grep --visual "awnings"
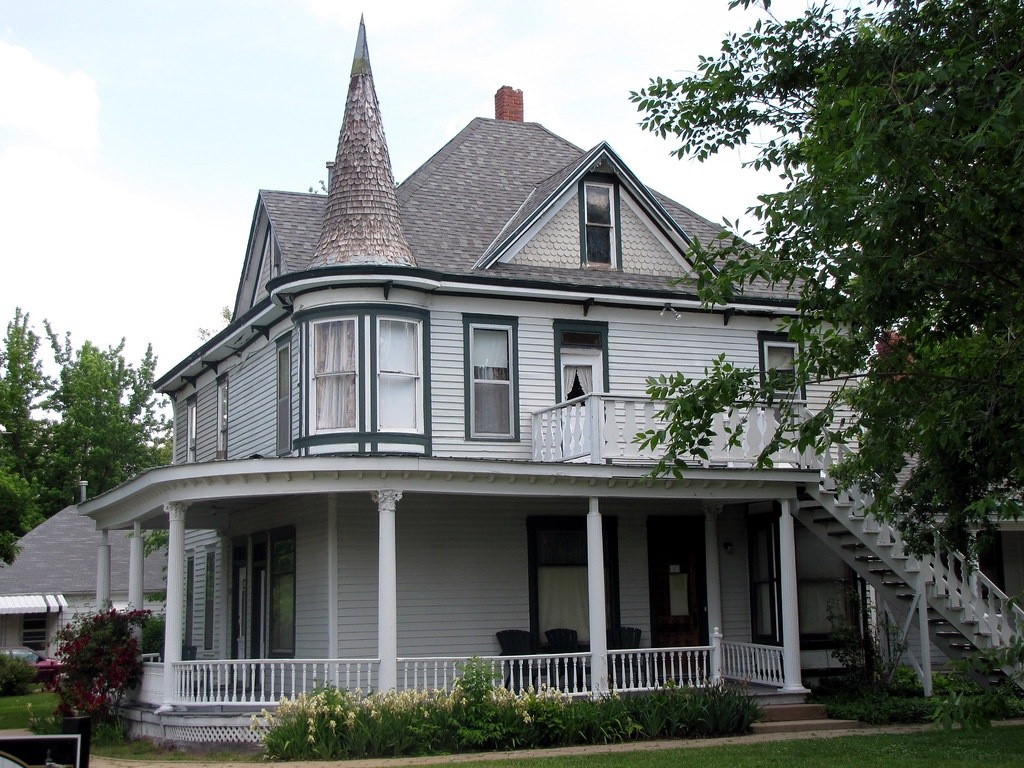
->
[0,595,67,614]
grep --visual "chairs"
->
[545,627,591,687]
[607,628,642,685]
[159,646,211,699]
[496,630,554,691]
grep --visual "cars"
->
[0,646,71,691]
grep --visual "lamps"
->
[665,303,682,321]
[722,542,733,554]
